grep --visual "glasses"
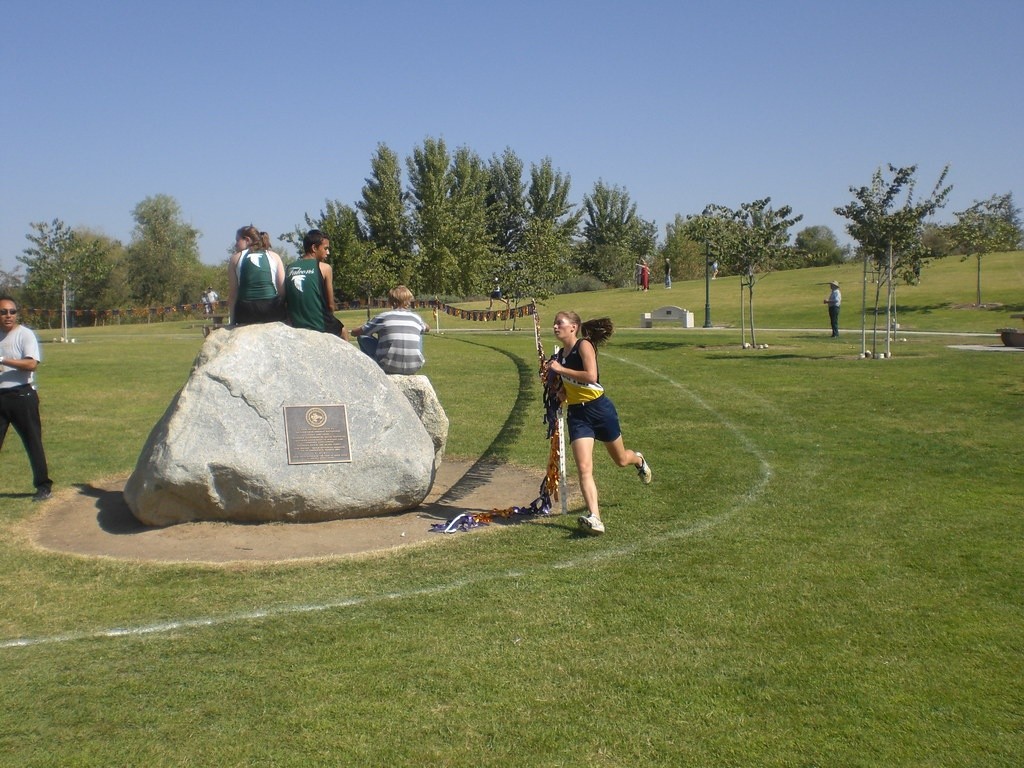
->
[0,309,17,315]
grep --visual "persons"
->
[284,230,348,341]
[910,259,923,284]
[351,285,430,375]
[0,297,53,500]
[824,281,841,338]
[712,260,718,279]
[203,287,218,314]
[485,273,508,309]
[223,226,286,325]
[636,258,649,292]
[664,258,671,289]
[544,310,651,535]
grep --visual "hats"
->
[828,280,840,287]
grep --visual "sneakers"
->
[635,452,651,484]
[578,516,605,535]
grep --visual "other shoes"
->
[642,288,648,291]
[665,286,671,289]
[32,484,52,502]
[831,333,840,338]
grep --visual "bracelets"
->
[0,356,3,363]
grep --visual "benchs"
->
[641,305,695,328]
